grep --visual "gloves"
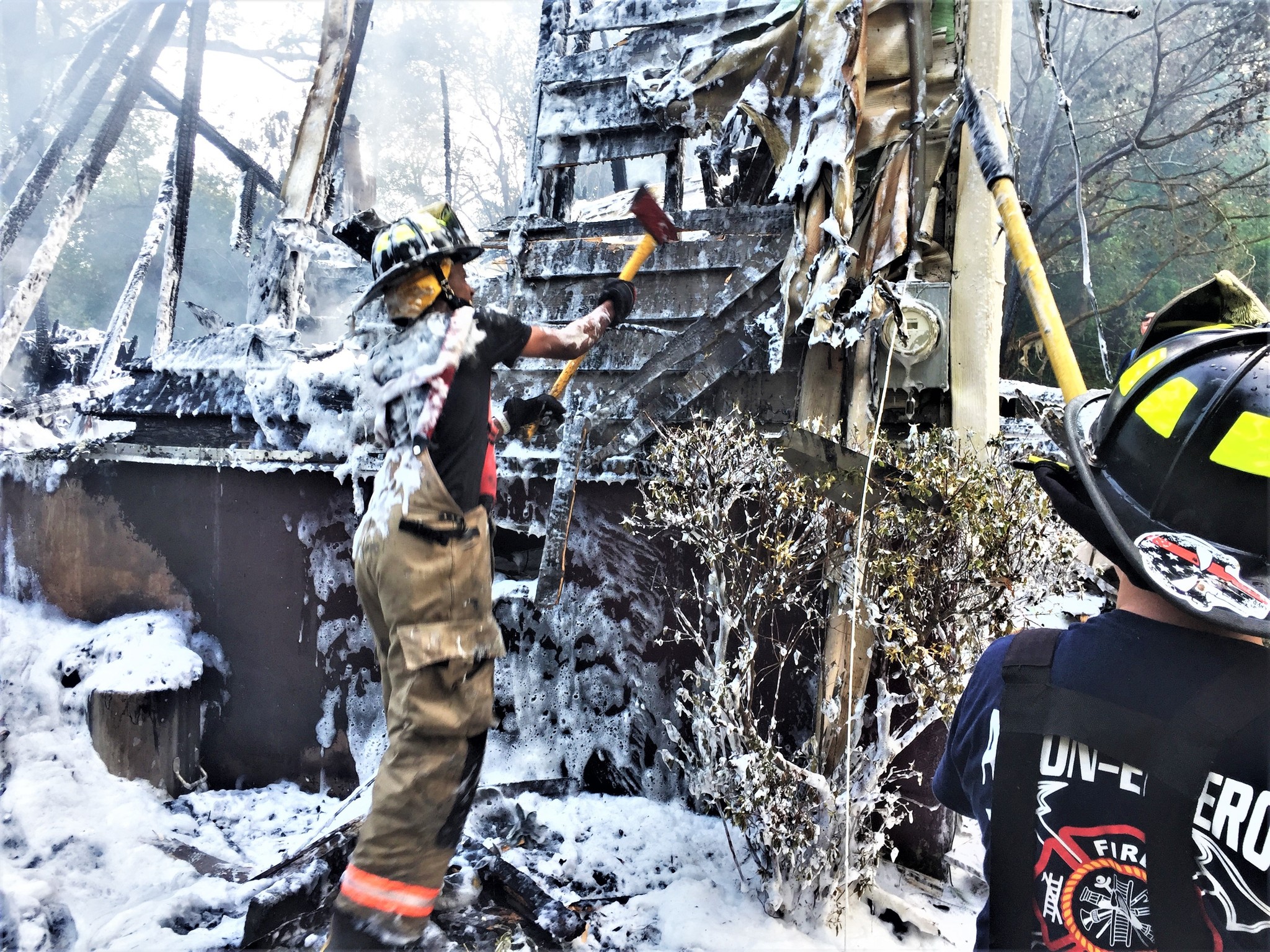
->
[503,394,566,435]
[595,277,637,328]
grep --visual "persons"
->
[329,214,640,952]
[934,312,1270,952]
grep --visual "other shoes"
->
[322,908,425,952]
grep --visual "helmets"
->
[1062,328,1269,640]
[355,210,486,314]
